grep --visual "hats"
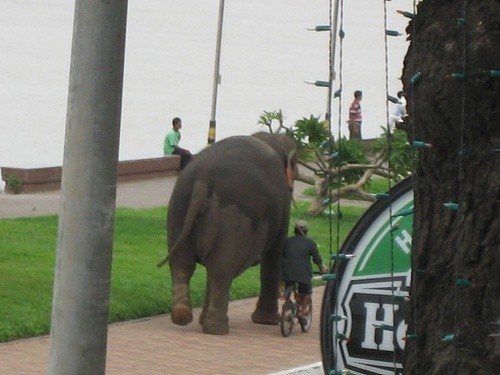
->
[295,220,309,230]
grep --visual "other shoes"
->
[297,314,307,325]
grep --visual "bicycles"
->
[280,279,313,338]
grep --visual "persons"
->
[388,93,407,132]
[164,118,191,170]
[349,91,363,140]
[283,221,327,323]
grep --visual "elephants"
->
[157,131,300,335]
